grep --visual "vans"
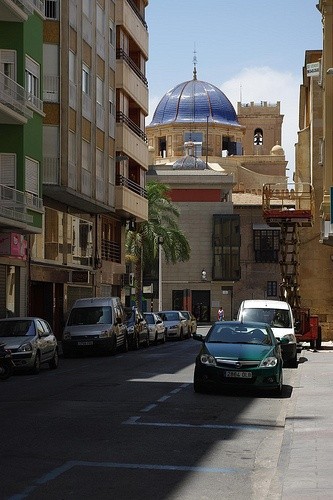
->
[61,296,129,356]
[234,299,300,364]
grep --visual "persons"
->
[218,307,224,321]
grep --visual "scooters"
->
[0,342,17,382]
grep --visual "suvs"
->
[123,306,150,351]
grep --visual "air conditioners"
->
[123,272,135,286]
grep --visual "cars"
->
[192,320,291,396]
[180,310,198,337]
[156,310,189,341]
[143,312,166,346]
[0,316,59,375]
